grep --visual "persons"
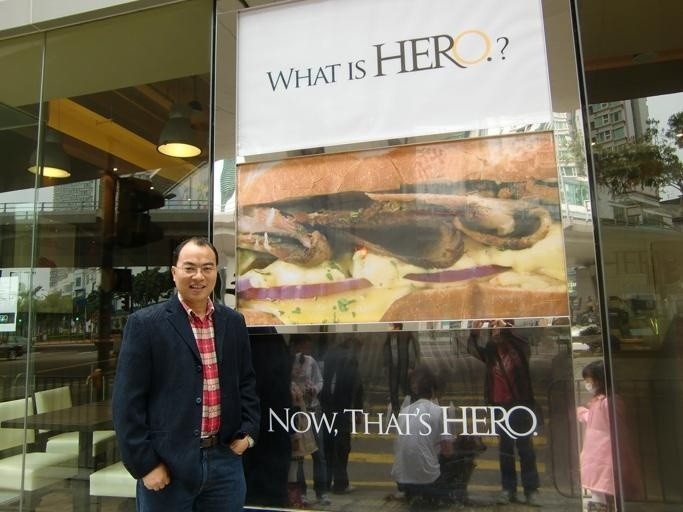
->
[110,237,262,512]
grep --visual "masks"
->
[585,382,599,395]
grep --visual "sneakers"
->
[317,494,330,504]
[527,492,544,507]
[498,490,516,504]
[300,495,310,505]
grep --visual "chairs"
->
[0,385,138,512]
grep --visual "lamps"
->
[156,76,202,159]
[26,99,71,177]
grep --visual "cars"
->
[571,294,659,354]
[0,335,32,361]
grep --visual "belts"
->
[199,436,219,448]
